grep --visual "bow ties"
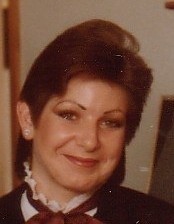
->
[24,188,103,224]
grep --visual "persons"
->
[0,18,174,224]
